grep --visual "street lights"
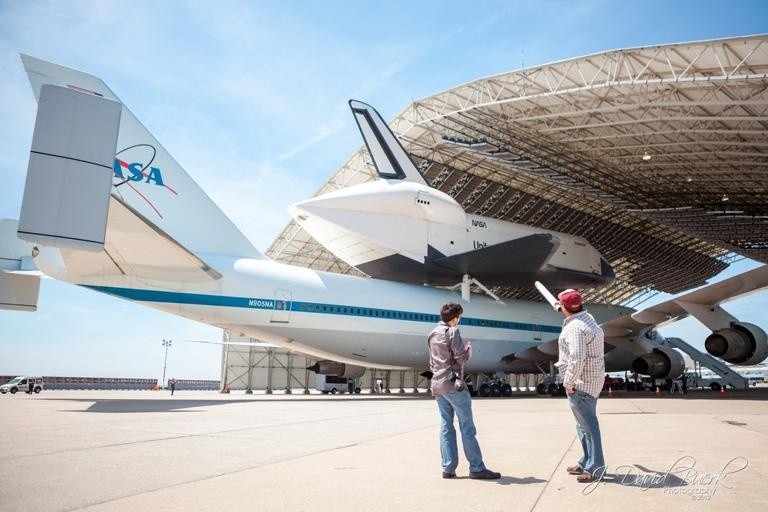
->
[161,339,172,389]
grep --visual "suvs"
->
[1,375,44,394]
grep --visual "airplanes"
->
[1,51,767,398]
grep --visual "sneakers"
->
[443,472,457,478]
[469,469,501,479]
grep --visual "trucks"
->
[316,372,361,394]
[674,373,729,392]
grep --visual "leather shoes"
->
[567,466,600,483]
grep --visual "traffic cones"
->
[607,387,614,398]
[719,391,729,398]
[656,387,663,397]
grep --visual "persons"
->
[676,373,690,395]
[426,301,501,479]
[380,382,384,391]
[554,289,606,482]
[169,377,176,395]
[29,383,34,394]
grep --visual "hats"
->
[558,288,584,310]
